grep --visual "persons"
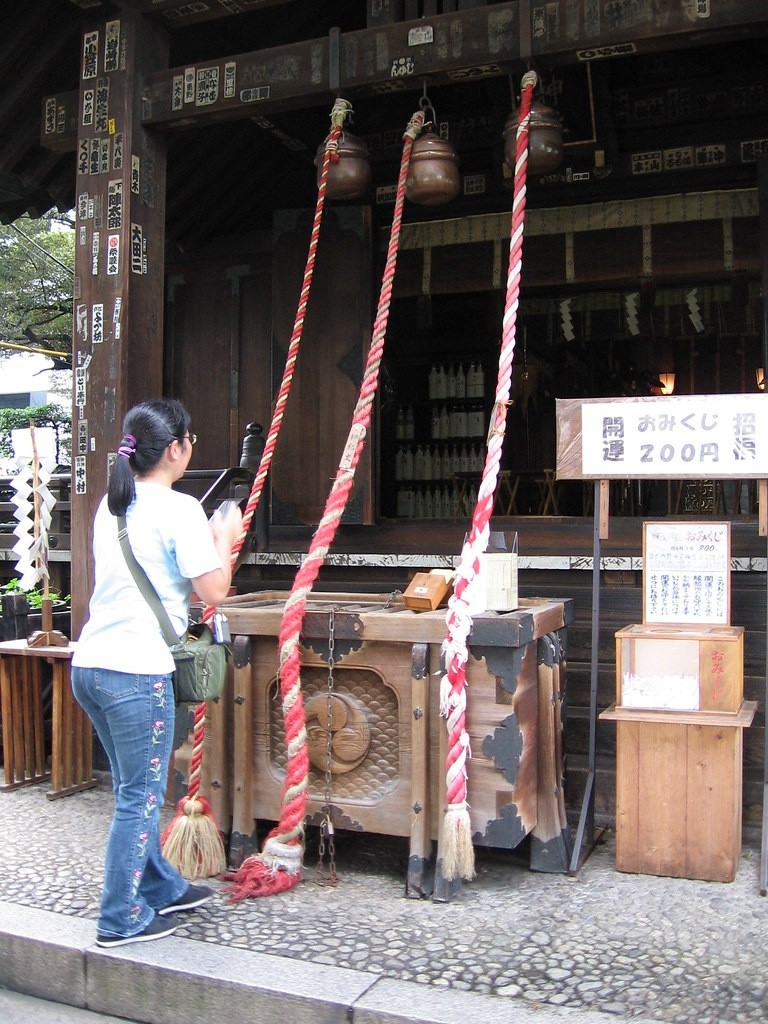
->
[71,399,244,948]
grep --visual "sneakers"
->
[156,884,214,914]
[95,914,178,947]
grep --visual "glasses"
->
[168,433,197,447]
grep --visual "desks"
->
[200,589,576,902]
[0,639,99,801]
[598,698,758,884]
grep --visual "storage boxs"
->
[614,624,745,717]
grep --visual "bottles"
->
[389,362,501,521]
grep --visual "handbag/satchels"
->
[169,623,232,707]
